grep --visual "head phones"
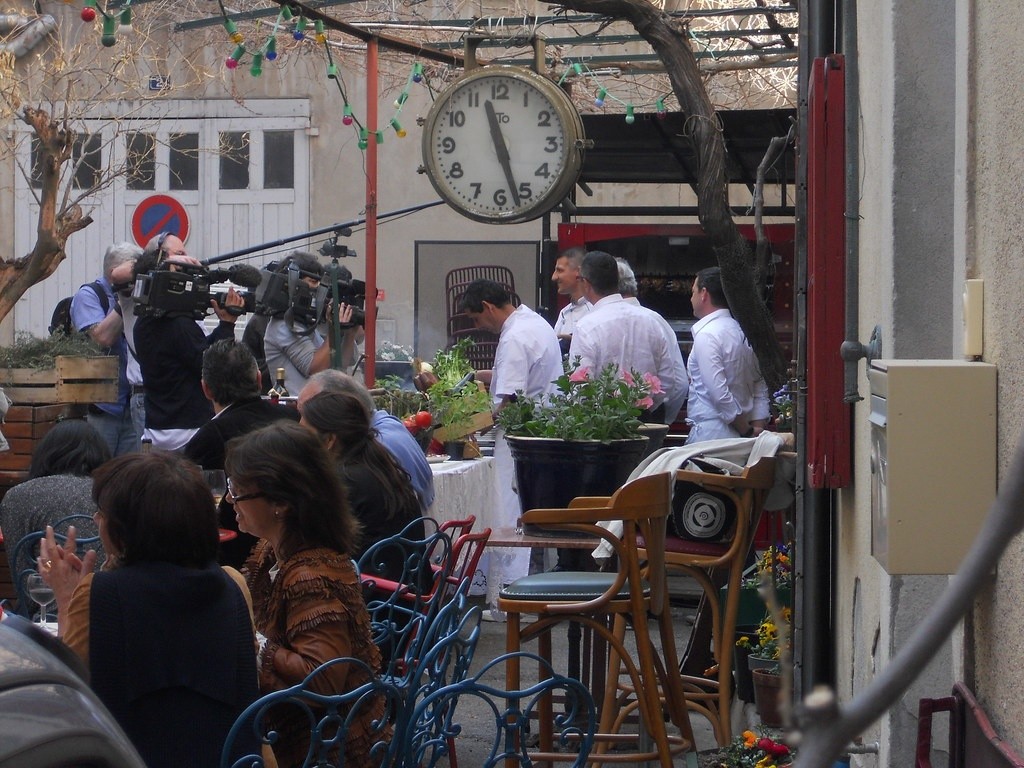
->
[151,231,174,267]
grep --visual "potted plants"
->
[433,381,490,461]
[505,354,647,536]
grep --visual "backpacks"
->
[48,282,110,342]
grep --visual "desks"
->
[423,456,506,621]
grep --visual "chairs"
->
[499,459,775,768]
[446,265,522,369]
[220,514,595,768]
[0,514,102,622]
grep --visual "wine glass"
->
[26,573,58,632]
[202,469,227,537]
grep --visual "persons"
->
[459,249,770,469]
[1,231,434,768]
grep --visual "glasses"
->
[576,275,588,283]
[226,477,275,504]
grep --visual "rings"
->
[43,560,52,573]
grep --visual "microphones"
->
[561,198,576,216]
[218,264,262,288]
[337,279,379,300]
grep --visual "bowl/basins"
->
[407,425,435,454]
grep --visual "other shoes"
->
[482,608,538,623]
[592,614,633,630]
[686,615,695,624]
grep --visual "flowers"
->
[721,543,794,765]
[571,363,665,409]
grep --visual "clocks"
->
[421,34,588,224]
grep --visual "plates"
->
[426,454,451,462]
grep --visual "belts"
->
[135,386,143,394]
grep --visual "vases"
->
[634,418,671,454]
[722,561,794,725]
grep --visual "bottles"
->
[272,368,289,397]
